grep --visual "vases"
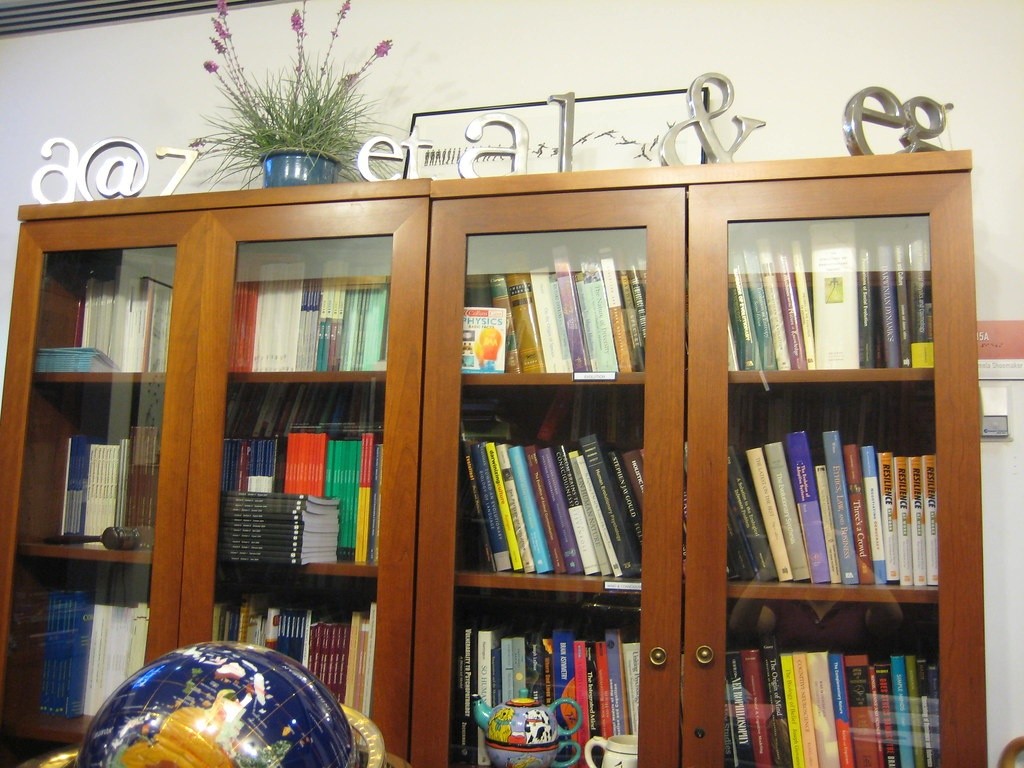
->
[260,149,341,188]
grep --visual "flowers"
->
[188,0,430,188]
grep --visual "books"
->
[26,220,939,768]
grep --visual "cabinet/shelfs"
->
[0,151,989,768]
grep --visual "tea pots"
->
[470,688,583,768]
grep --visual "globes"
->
[17,640,386,768]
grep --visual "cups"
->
[584,735,638,768]
